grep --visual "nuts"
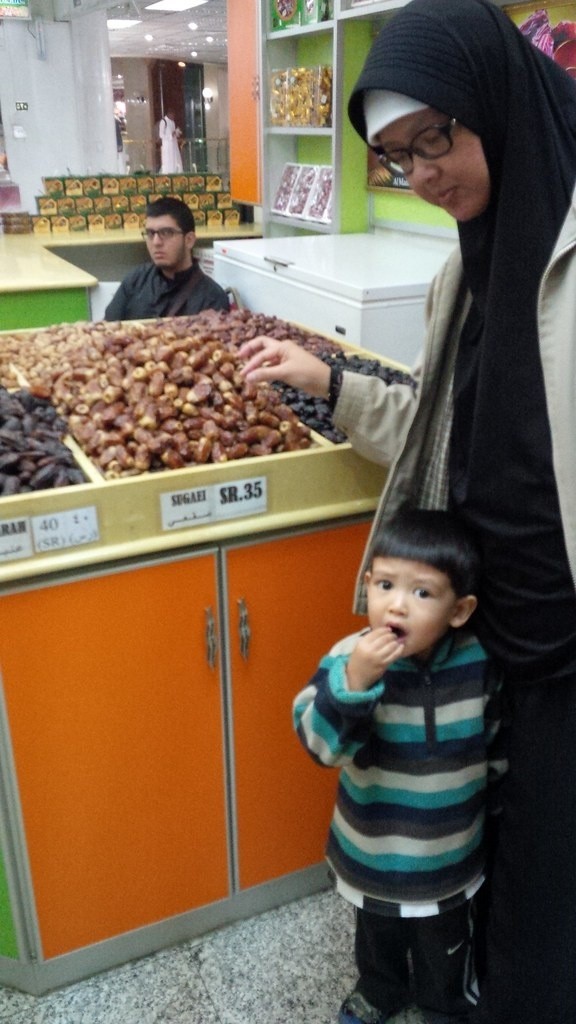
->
[0,309,419,510]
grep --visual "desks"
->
[0,223,262,331]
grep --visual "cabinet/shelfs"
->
[1,514,376,999]
[258,0,576,239]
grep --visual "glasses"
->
[142,228,184,242]
[377,117,458,177]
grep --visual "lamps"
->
[203,88,214,103]
[133,90,145,104]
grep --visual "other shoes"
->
[338,990,385,1024]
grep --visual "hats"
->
[365,91,430,148]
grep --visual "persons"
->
[158,108,185,174]
[291,511,510,1024]
[237,0,576,1024]
[103,197,230,322]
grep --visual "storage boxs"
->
[272,162,335,224]
[0,312,419,578]
